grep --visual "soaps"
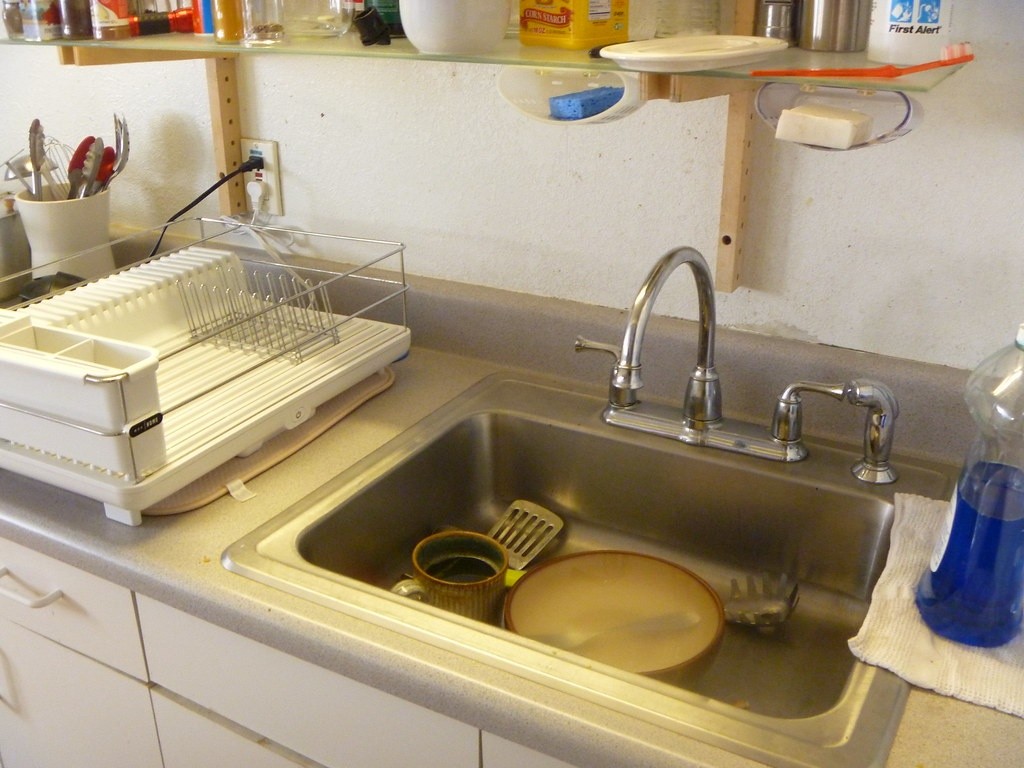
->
[771,100,878,153]
[550,86,635,126]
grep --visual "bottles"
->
[3,1,407,46]
[915,322,1023,648]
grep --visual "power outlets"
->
[240,137,283,218]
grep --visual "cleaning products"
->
[517,0,634,53]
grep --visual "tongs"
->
[67,136,115,200]
[29,118,105,199]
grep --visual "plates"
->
[600,34,788,71]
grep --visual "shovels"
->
[484,499,565,571]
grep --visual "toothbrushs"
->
[744,36,980,83]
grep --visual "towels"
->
[842,487,1024,723]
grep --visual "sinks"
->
[220,365,942,766]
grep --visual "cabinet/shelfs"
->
[2,535,575,766]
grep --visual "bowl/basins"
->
[399,0,512,56]
[501,549,724,690]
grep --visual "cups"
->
[794,0,874,53]
[391,528,510,630]
[864,0,954,64]
[15,181,115,283]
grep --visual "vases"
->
[397,0,511,56]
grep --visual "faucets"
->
[609,244,725,445]
